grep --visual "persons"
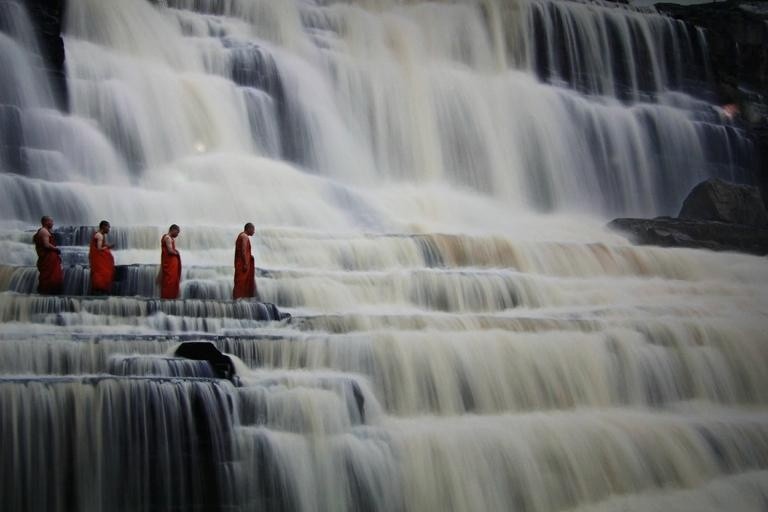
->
[159,224,183,299]
[87,220,116,296]
[33,215,65,295]
[232,222,257,300]
